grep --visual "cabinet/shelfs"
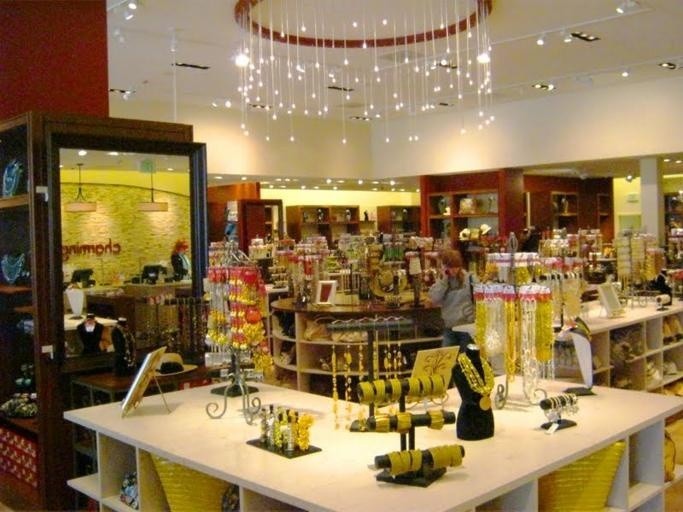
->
[62,374,683,510]
[453,296,682,402]
[425,189,505,245]
[378,206,422,237]
[264,204,275,239]
[2,111,214,509]
[524,191,614,251]
[287,204,359,240]
[270,295,444,401]
[664,193,683,260]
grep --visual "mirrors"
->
[43,136,204,492]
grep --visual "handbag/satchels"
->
[304,316,337,341]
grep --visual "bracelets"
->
[354,375,445,433]
[258,409,313,450]
[386,445,463,476]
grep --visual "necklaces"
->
[1,253,24,281]
[3,163,20,193]
[206,266,274,375]
[332,320,403,428]
[511,237,583,320]
[459,282,556,411]
[116,322,136,366]
[616,234,665,282]
